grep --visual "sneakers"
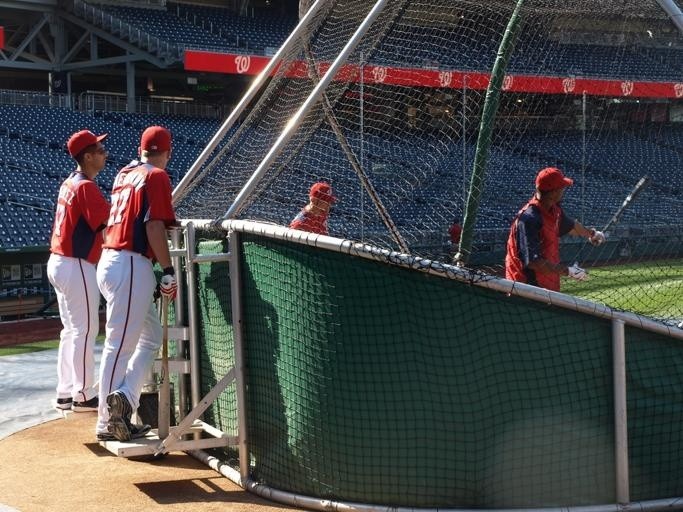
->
[97,424,152,440]
[56,397,99,412]
[106,390,132,441]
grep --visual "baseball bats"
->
[601,176,649,233]
[158,297,170,439]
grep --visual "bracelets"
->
[165,266,174,275]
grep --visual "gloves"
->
[589,228,606,246]
[159,266,177,304]
[567,261,589,282]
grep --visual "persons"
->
[506,167,607,293]
[47,130,112,413]
[290,182,337,235]
[447,219,463,257]
[95,125,177,442]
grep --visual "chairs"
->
[2,1,682,256]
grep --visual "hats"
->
[67,131,109,158]
[536,167,573,192]
[310,182,336,202]
[141,126,172,152]
